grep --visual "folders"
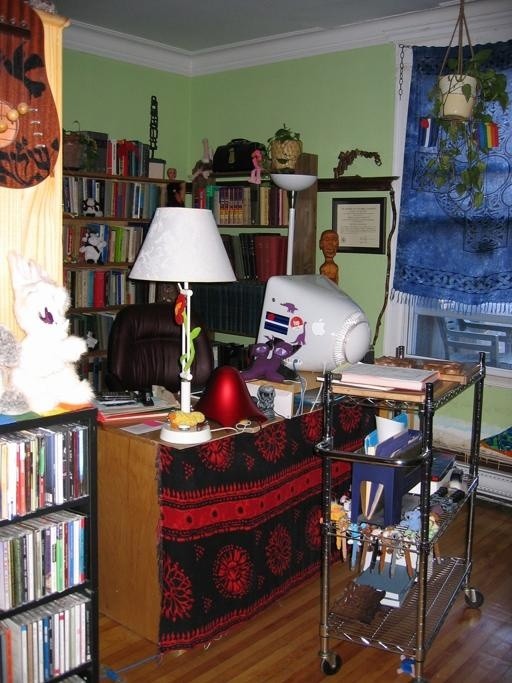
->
[350,413,423,521]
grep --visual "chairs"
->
[436,316,512,370]
[106,302,213,392]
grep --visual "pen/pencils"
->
[390,533,399,579]
[429,520,433,576]
[404,533,414,578]
[429,515,442,564]
[341,517,347,563]
[379,542,386,576]
[427,554,431,583]
[350,523,358,573]
[335,520,341,550]
[370,538,379,574]
[360,524,371,573]
[414,530,424,584]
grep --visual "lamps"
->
[128,207,238,444]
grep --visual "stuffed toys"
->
[0,248,95,414]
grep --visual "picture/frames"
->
[332,197,387,254]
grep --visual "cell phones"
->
[101,392,131,400]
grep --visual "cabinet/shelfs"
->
[192,153,318,349]
[315,346,486,683]
[63,169,186,393]
[0,7,71,391]
[0,409,98,683]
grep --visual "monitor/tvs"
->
[254,275,371,373]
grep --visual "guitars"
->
[0,1,62,190]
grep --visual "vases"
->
[415,48,509,209]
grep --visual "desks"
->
[98,393,321,645]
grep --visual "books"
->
[208,340,253,370]
[64,226,145,268]
[69,310,119,393]
[220,235,287,285]
[317,360,395,391]
[62,175,165,222]
[191,285,264,337]
[183,186,289,230]
[63,269,157,305]
[1,424,89,519]
[356,559,419,609]
[1,593,90,682]
[108,138,149,178]
[2,510,88,609]
[327,361,441,391]
